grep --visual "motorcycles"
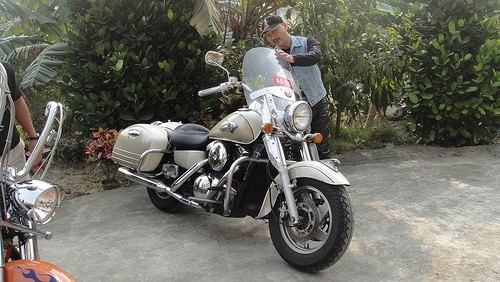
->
[0,100,77,282]
[111,45,355,273]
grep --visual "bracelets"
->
[29,133,39,140]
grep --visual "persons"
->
[260,15,332,162]
[0,61,43,183]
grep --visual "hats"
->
[261,15,283,37]
